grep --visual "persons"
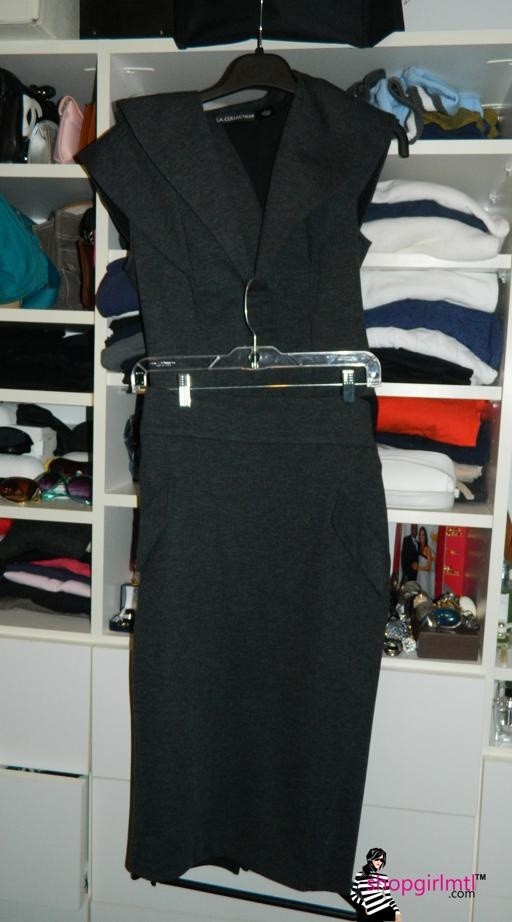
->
[412,526,433,598]
[398,523,420,584]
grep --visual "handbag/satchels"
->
[54,94,85,170]
[0,192,62,309]
[31,207,84,311]
[27,118,60,164]
[76,228,96,310]
[78,100,97,152]
[0,67,60,164]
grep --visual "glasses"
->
[374,858,385,862]
[0,476,39,503]
[46,456,93,478]
[37,471,93,505]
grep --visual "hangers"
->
[80,0,415,170]
[124,278,383,400]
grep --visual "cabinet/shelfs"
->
[0,35,512,922]
